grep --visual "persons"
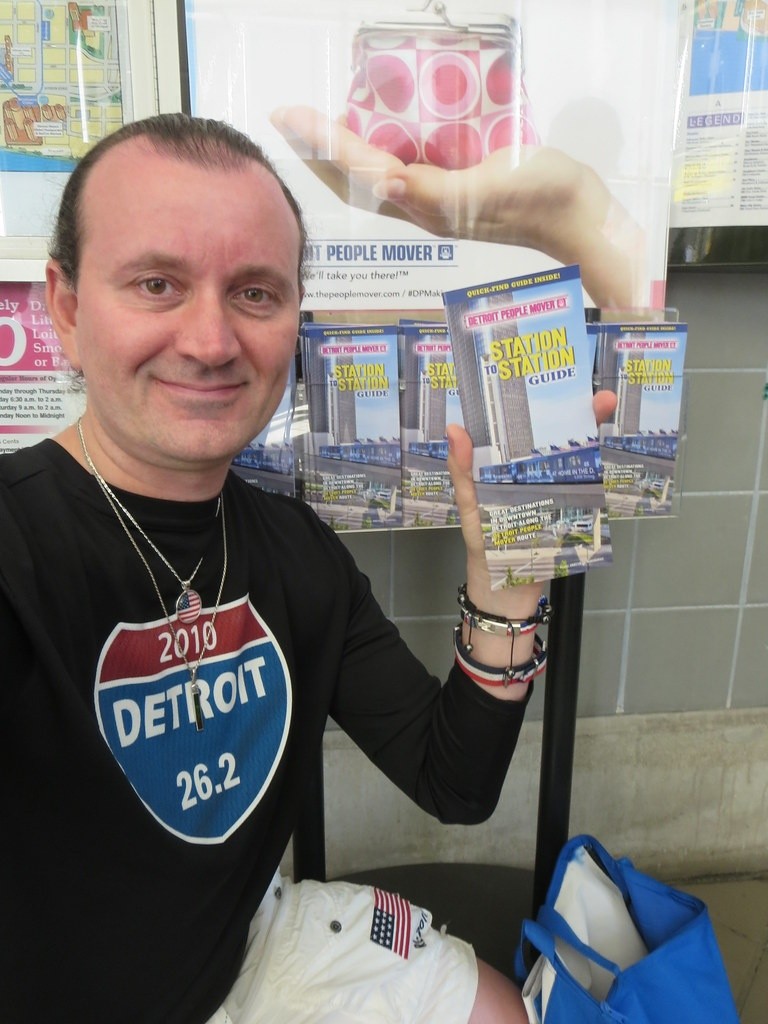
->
[0,114,556,1024]
[266,104,651,318]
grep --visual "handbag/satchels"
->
[514,833,740,1024]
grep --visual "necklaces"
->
[77,418,228,731]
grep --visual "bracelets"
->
[457,582,553,638]
[452,624,547,688]
